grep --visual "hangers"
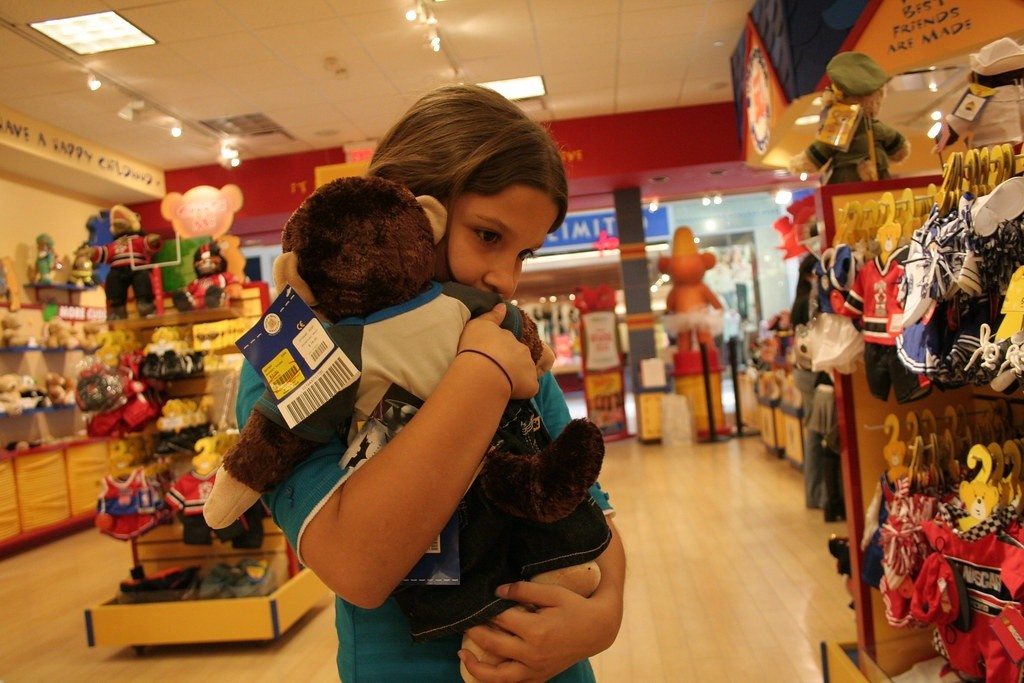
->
[877,399,1024,532]
[831,145,1018,261]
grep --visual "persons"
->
[790,253,857,520]
[234,83,628,683]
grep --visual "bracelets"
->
[457,349,515,394]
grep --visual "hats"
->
[910,549,971,633]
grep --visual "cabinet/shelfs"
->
[83,280,327,657]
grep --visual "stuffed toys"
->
[202,169,611,683]
[784,36,1024,186]
[1,202,262,554]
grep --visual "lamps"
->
[649,172,808,213]
[87,72,240,169]
[928,122,943,145]
[406,1,440,52]
